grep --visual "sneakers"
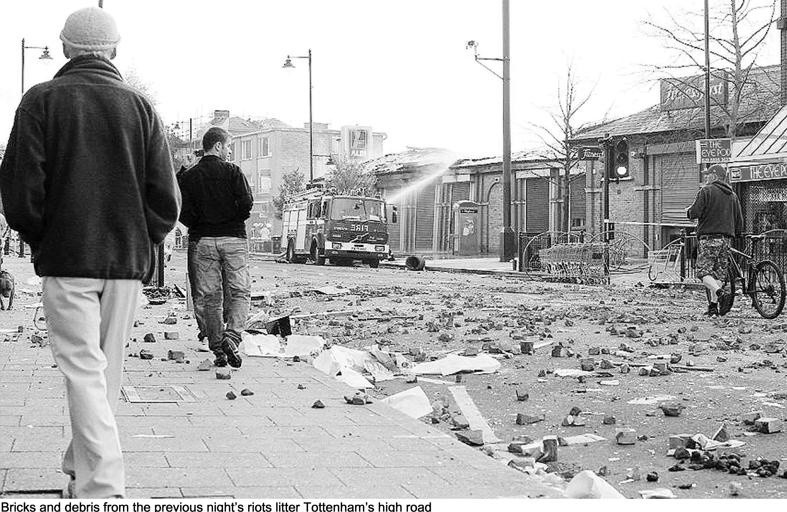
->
[708,288,733,319]
[212,336,243,368]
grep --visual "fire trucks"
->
[280,186,397,268]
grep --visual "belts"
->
[701,236,729,239]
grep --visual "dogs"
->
[0,271,16,311]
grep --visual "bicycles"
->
[705,234,786,320]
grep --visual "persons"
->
[1,6,183,497]
[181,126,255,369]
[684,165,744,319]
[173,155,211,352]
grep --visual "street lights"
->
[282,49,313,182]
[21,37,55,96]
[465,0,514,261]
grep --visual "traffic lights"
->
[612,137,629,178]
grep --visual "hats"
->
[701,164,726,177]
[59,6,122,51]
